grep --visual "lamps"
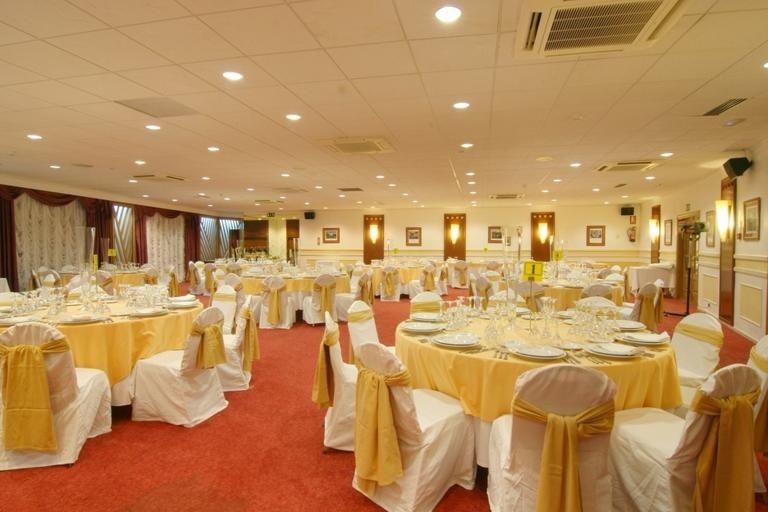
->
[451,223,460,245]
[714,201,732,242]
[370,225,378,245]
[648,219,658,244]
[539,222,547,244]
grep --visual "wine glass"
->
[120,263,140,271]
[15,286,168,315]
[439,296,622,339]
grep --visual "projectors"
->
[679,221,707,235]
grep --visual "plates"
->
[400,306,665,360]
[0,296,199,326]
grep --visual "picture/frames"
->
[488,226,503,244]
[323,228,340,243]
[406,227,422,246]
[743,197,761,241]
[664,220,672,246]
[586,225,605,246]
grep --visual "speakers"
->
[304,211,316,219]
[621,207,634,215]
[723,157,753,179]
